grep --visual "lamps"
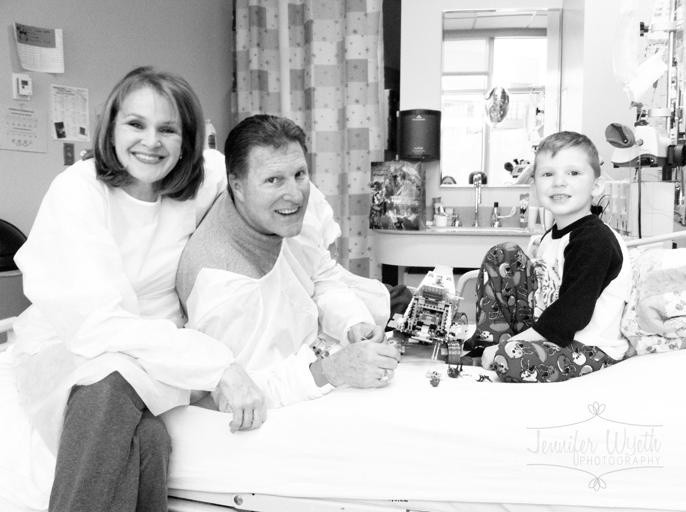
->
[395,108,441,227]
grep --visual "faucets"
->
[472,174,482,226]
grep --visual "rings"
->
[379,371,389,383]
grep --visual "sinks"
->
[437,227,520,234]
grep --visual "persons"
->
[385,263,467,364]
[173,113,402,412]
[10,65,341,512]
[442,175,456,184]
[462,130,632,383]
[469,172,488,184]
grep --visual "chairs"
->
[456,270,480,321]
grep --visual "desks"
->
[374,228,530,286]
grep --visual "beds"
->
[0,270,685,512]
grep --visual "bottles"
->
[432,196,442,215]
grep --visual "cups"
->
[433,215,447,227]
